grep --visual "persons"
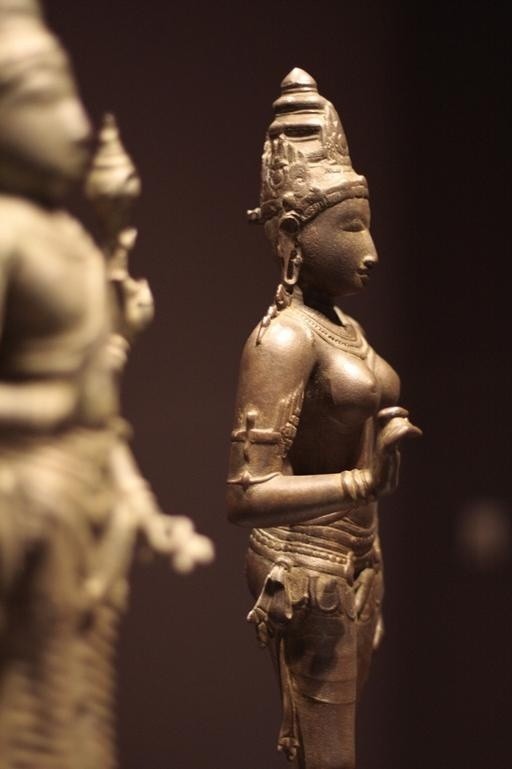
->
[1,0,217,769]
[224,66,424,769]
